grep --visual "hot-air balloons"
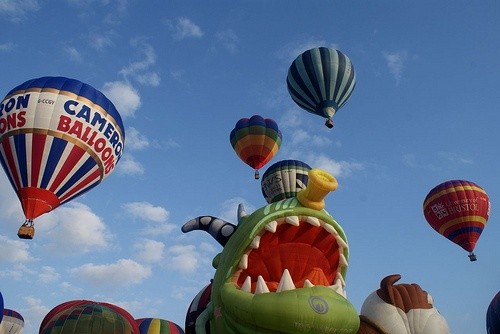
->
[423,179,490,261]
[261,159,313,205]
[0,75,126,242]
[38,298,140,334]
[229,114,283,180]
[286,47,356,129]
[0,308,25,334]
[135,317,184,334]
[185,279,212,334]
[486,290,500,334]
